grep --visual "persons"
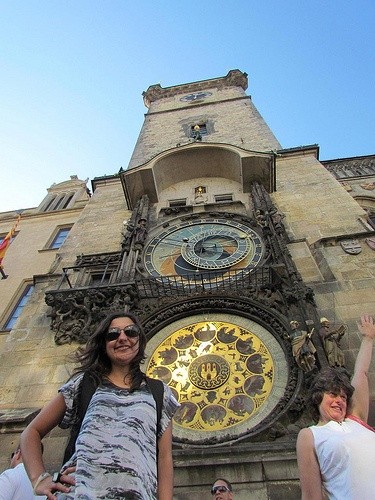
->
[121,220,147,246]
[18,313,182,500]
[64,296,109,341]
[0,442,48,500]
[296,315,375,500]
[251,207,286,240]
[283,317,348,376]
[210,478,233,500]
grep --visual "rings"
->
[52,472,63,483]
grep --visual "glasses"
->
[105,326,141,341]
[11,452,17,458]
[211,485,230,495]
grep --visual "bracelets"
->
[34,472,50,495]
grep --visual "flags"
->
[0,215,21,267]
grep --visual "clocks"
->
[143,217,264,291]
